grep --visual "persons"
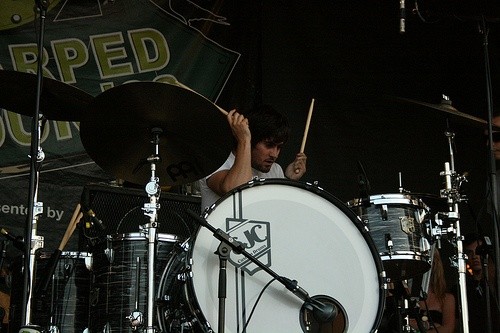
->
[462,241,487,333]
[200,104,308,214]
[482,114,500,254]
[409,250,455,333]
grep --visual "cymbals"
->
[0,68,83,122]
[394,94,490,128]
[79,82,233,185]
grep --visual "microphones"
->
[399,0,406,33]
[360,181,370,207]
[84,205,106,232]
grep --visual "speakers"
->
[78,184,202,251]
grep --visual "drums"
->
[104,233,180,331]
[344,193,432,282]
[29,251,95,333]
[156,177,388,333]
